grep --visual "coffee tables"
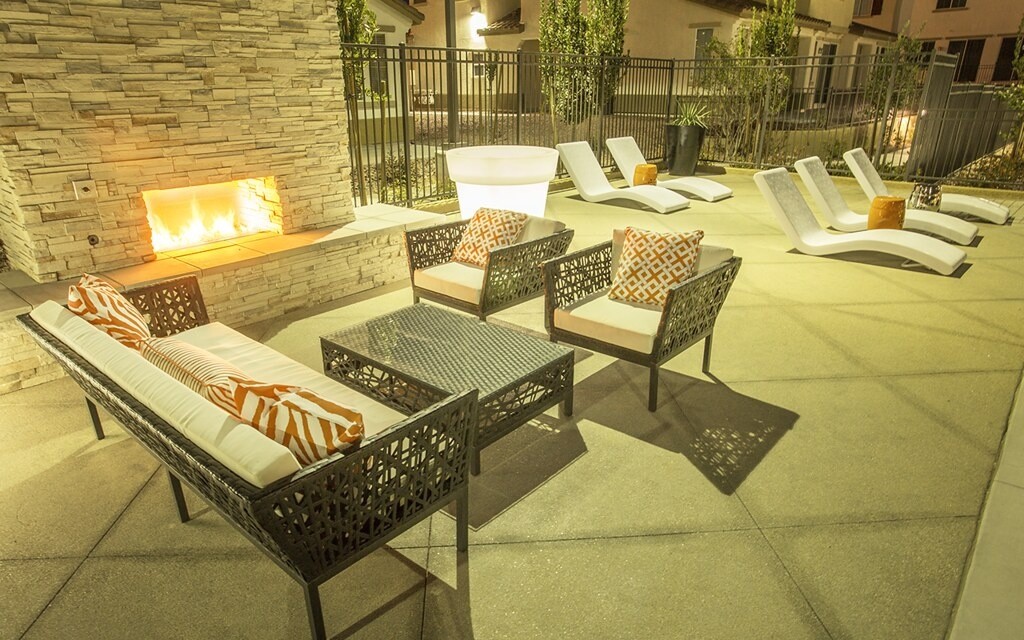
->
[318,302,574,477]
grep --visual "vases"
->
[447,145,559,218]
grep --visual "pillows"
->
[450,209,528,269]
[607,227,705,305]
[67,273,365,478]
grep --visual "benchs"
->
[17,274,479,640]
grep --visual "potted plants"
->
[665,97,711,177]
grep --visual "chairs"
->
[403,211,574,322]
[556,135,733,212]
[539,228,742,411]
[753,147,1009,276]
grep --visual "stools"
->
[867,195,906,230]
[907,180,942,213]
[633,164,658,187]
